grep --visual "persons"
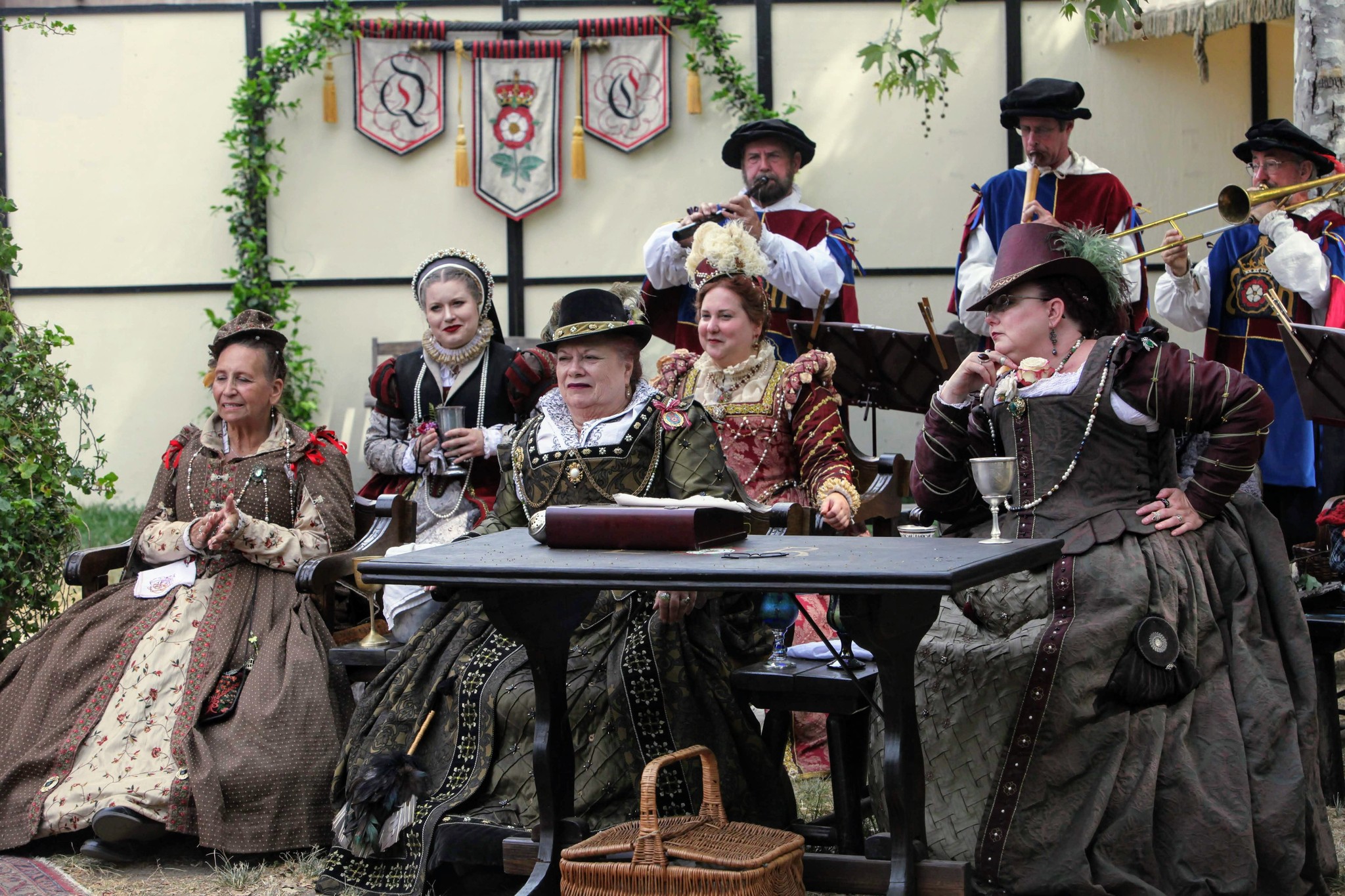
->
[1154,118,1345,489]
[946,77,1149,351]
[314,281,798,896]
[909,222,1345,896]
[357,246,558,546]
[647,216,861,780]
[636,118,866,356]
[0,309,356,865]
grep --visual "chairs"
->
[61,337,909,890]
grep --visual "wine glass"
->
[351,556,390,647]
[435,406,469,476]
[969,457,1017,544]
[760,590,799,669]
[827,593,866,669]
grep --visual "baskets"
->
[1293,495,1345,583]
[559,746,805,896]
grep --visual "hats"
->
[683,219,759,294]
[209,309,288,362]
[999,78,1092,131]
[410,248,494,326]
[722,119,816,170]
[1233,118,1336,176]
[965,222,1113,327]
[535,282,652,351]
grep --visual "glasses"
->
[1245,159,1298,178]
[985,292,1066,318]
[1014,121,1070,137]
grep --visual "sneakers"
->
[314,877,346,895]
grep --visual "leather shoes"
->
[80,838,140,865]
[90,804,165,842]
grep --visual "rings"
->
[658,593,670,600]
[984,349,992,353]
[1000,356,1007,366]
[679,597,690,604]
[1151,511,1160,521]
[227,521,235,533]
[1160,498,1169,508]
[977,353,990,365]
[199,525,203,534]
[1175,515,1182,524]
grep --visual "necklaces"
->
[1055,336,1085,372]
[229,445,241,458]
[701,357,769,405]
[413,343,490,518]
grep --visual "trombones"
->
[1104,168,1345,269]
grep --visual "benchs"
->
[732,649,878,856]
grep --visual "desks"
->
[356,528,1065,896]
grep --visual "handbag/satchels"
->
[544,500,751,551]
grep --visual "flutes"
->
[1021,147,1046,223]
[670,172,768,241]
